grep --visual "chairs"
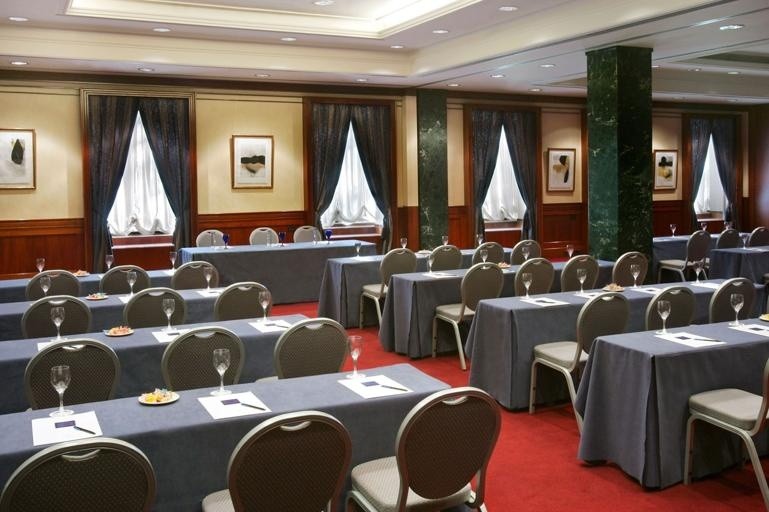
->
[351,385,505,512]
[682,350,769,510]
[0,437,162,511]
[203,410,355,512]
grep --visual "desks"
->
[1,363,455,512]
[590,317,768,486]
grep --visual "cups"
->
[265,230,275,247]
[311,227,319,245]
[209,232,216,249]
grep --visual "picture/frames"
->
[654,150,678,191]
[546,147,575,192]
[1,126,37,191]
[231,134,273,189]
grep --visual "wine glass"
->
[629,265,640,289]
[346,336,366,379]
[161,299,177,331]
[476,233,483,245]
[480,249,488,269]
[256,291,271,322]
[169,252,177,271]
[567,245,574,259]
[105,255,114,271]
[655,300,672,334]
[521,273,533,299]
[400,238,407,252]
[36,258,45,273]
[48,366,74,417]
[670,224,676,237]
[50,307,66,342]
[354,242,362,259]
[522,247,531,261]
[427,254,435,273]
[278,231,287,248]
[442,236,449,250]
[221,233,230,250]
[326,230,333,244]
[40,276,51,297]
[692,262,704,284]
[576,268,587,293]
[729,294,745,326]
[701,223,733,231]
[203,267,213,290]
[211,349,231,395]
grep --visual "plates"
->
[85,295,109,300]
[496,263,511,268]
[74,273,90,276]
[603,286,625,292]
[138,394,180,405]
[105,330,134,336]
